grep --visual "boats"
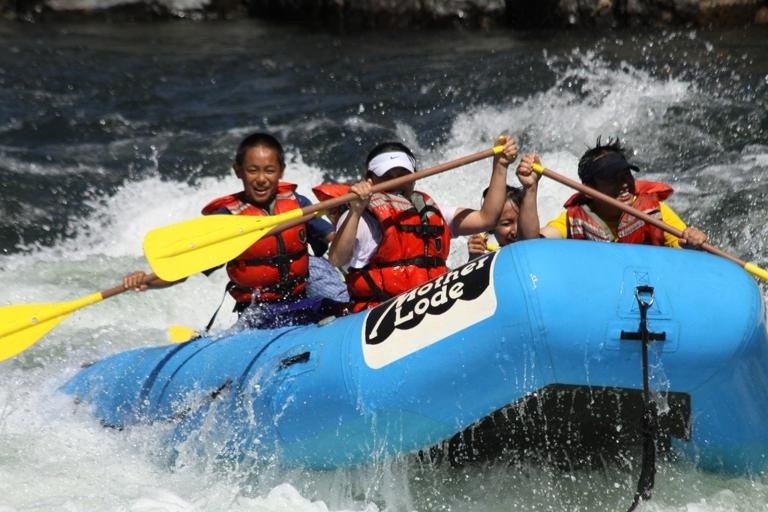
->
[43,231,768,487]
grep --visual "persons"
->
[512,134,709,252]
[467,182,524,264]
[327,133,517,314]
[121,133,337,331]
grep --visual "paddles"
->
[145,135,506,282]
[0,273,157,360]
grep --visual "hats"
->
[577,149,641,182]
[365,150,418,178]
